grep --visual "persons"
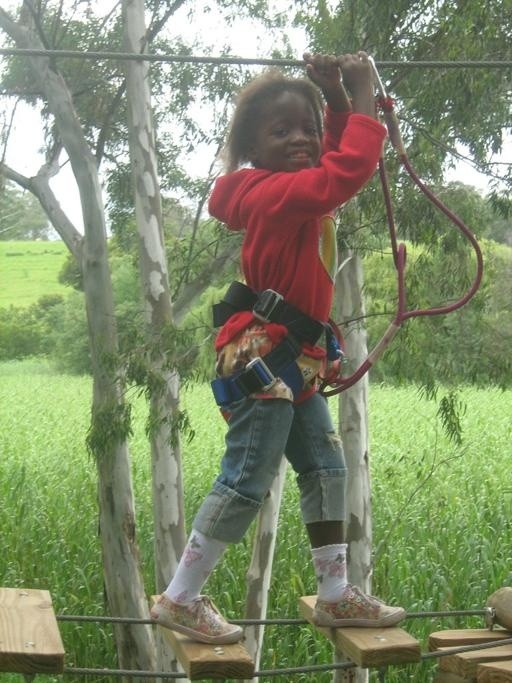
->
[149,50,406,645]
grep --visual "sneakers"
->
[312,583,407,628]
[150,592,245,646]
